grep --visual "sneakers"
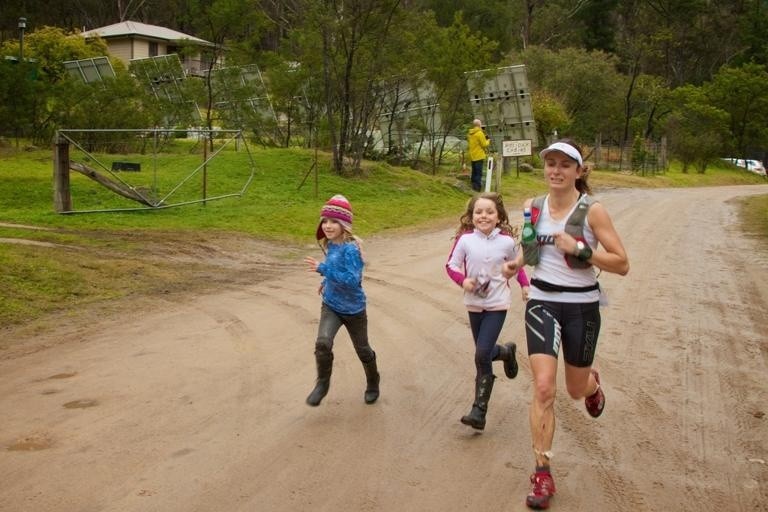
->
[584,369,606,419]
[525,470,557,510]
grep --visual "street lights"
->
[18,17,27,58]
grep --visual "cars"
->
[723,158,766,176]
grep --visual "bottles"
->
[475,269,489,298]
[520,209,541,266]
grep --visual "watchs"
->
[576,239,585,256]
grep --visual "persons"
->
[445,192,530,430]
[499,137,629,510]
[304,193,380,406]
[468,120,491,190]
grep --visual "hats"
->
[538,142,583,168]
[315,194,354,240]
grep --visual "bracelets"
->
[578,245,592,262]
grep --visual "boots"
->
[461,374,497,431]
[360,350,381,404]
[492,342,519,380]
[306,353,334,407]
